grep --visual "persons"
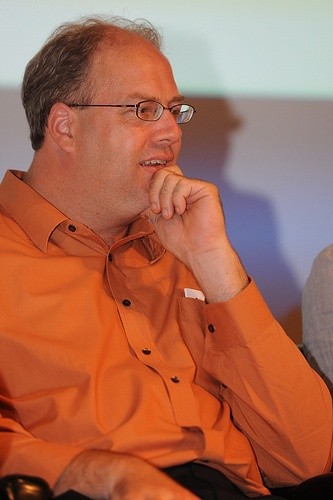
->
[1,17,333,500]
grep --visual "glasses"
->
[68,99,197,124]
